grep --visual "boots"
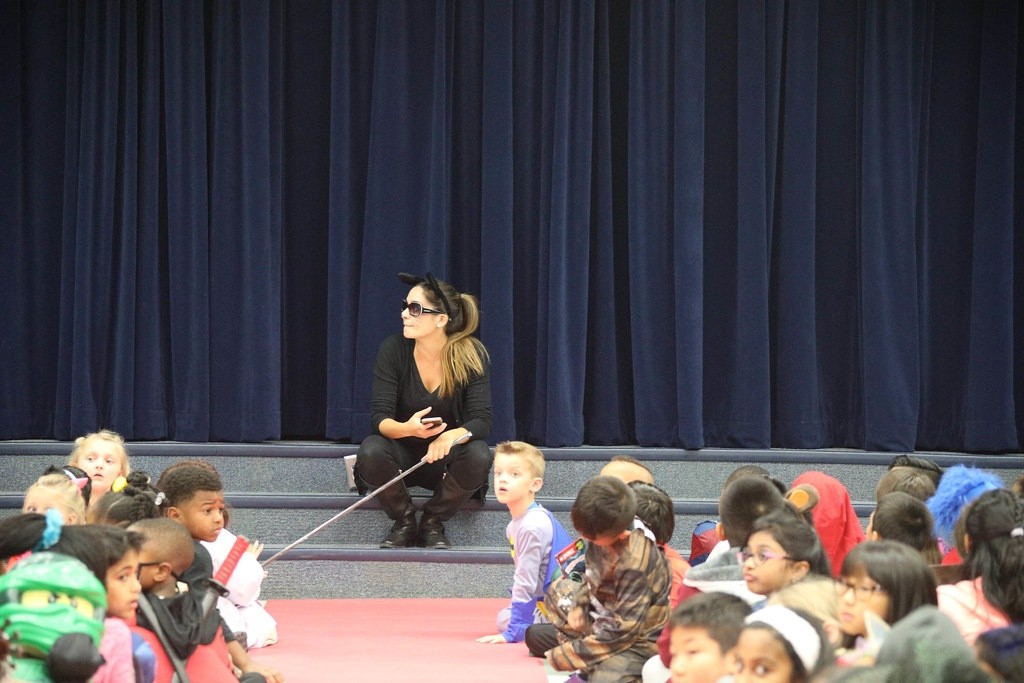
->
[358,468,418,549]
[417,462,484,549]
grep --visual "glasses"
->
[833,575,892,602]
[737,549,801,568]
[402,299,451,322]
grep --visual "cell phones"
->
[422,417,442,429]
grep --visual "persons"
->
[352,273,495,548]
[523,453,1023,683]
[0,430,278,683]
[474,439,573,643]
